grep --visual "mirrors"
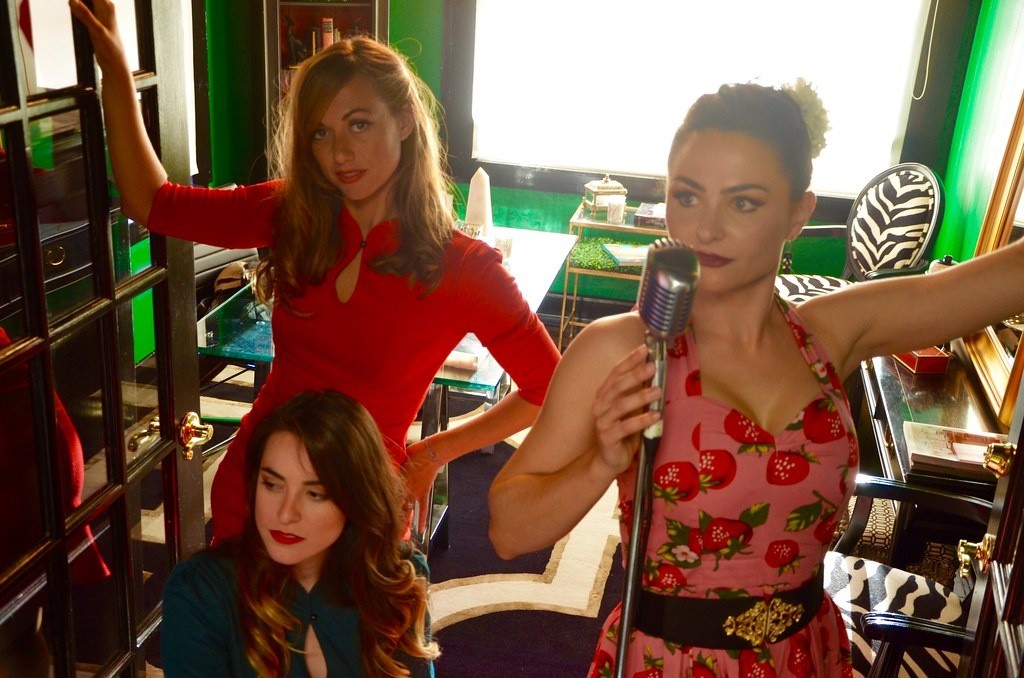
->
[961,93,1024,438]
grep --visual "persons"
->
[486,80,1024,678]
[0,140,121,678]
[70,1,563,546]
[163,388,444,678]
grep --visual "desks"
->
[196,223,580,578]
[852,351,999,564]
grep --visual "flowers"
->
[780,77,831,159]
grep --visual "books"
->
[901,419,1010,479]
[602,243,654,267]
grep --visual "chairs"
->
[797,160,942,425]
[824,473,993,678]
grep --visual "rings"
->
[407,501,414,510]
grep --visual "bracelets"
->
[424,435,446,467]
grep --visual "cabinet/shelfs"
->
[557,201,669,357]
[262,0,391,185]
[0,165,142,611]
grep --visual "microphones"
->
[635,238,700,338]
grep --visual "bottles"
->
[924,255,959,274]
